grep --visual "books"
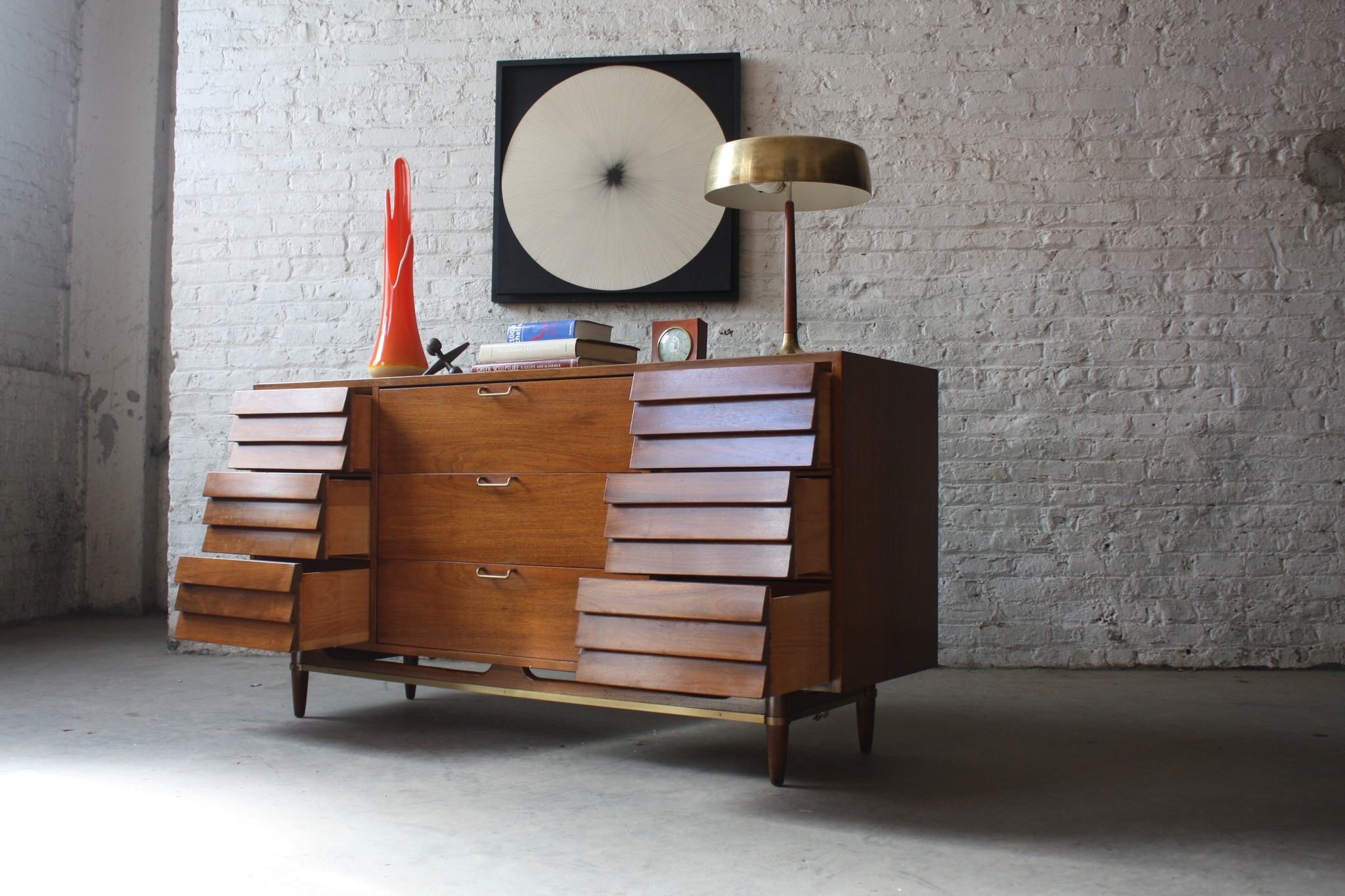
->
[470,320,641,373]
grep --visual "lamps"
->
[702,136,872,355]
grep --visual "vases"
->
[369,157,428,377]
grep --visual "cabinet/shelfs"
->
[173,350,939,789]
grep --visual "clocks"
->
[650,318,708,363]
